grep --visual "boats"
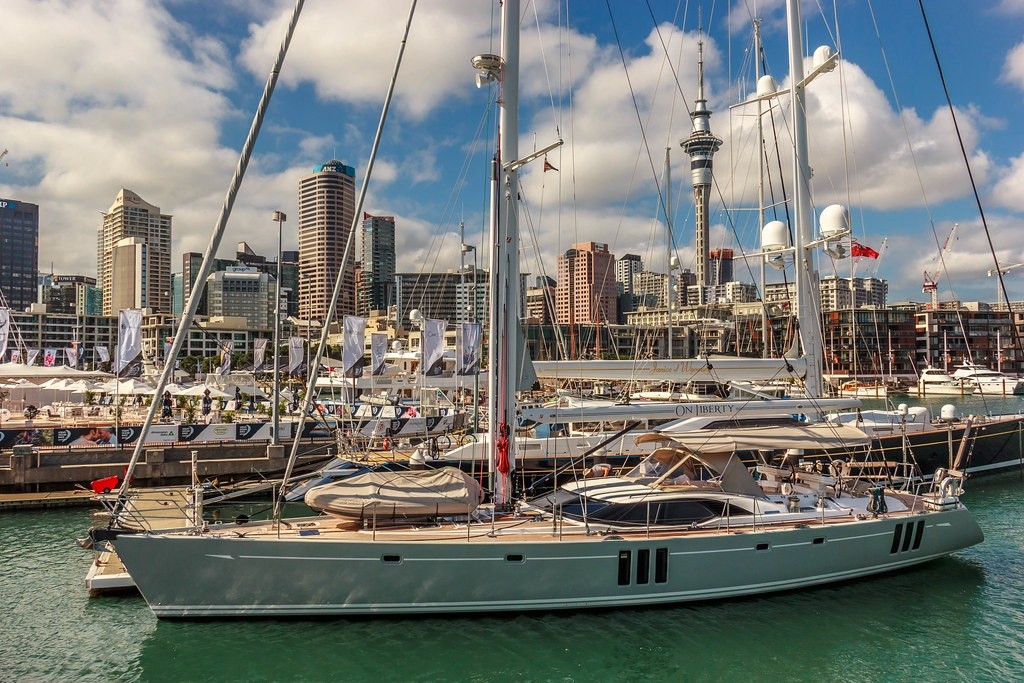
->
[303,464,485,525]
[282,326,1024,509]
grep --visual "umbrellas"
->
[0,377,232,405]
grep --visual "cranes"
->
[986,260,1024,312]
[866,237,889,277]
[922,222,960,308]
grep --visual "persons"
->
[583,463,613,478]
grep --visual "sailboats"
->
[85,0,985,623]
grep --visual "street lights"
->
[270,210,290,446]
[164,290,175,383]
[460,242,480,433]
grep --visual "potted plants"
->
[23,404,41,427]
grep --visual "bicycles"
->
[434,421,477,452]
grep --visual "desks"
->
[173,408,196,420]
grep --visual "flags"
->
[851,241,879,259]
[544,160,558,173]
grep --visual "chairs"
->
[88,407,101,418]
[71,408,85,422]
[47,409,60,421]
